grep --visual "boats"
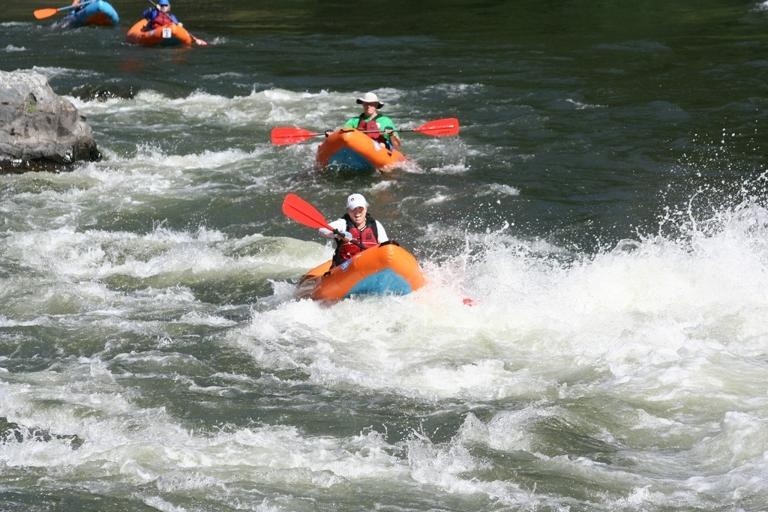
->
[315,128,411,180]
[126,18,192,46]
[294,240,429,304]
[52,0,120,33]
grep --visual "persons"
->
[317,193,389,271]
[71,0,101,14]
[141,0,183,33]
[324,92,401,152]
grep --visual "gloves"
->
[335,230,353,243]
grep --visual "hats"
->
[356,91,384,109]
[346,194,368,211]
[159,0,170,6]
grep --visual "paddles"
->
[282,193,367,252]
[148,0,207,46]
[271,117,460,146]
[33,0,92,20]
